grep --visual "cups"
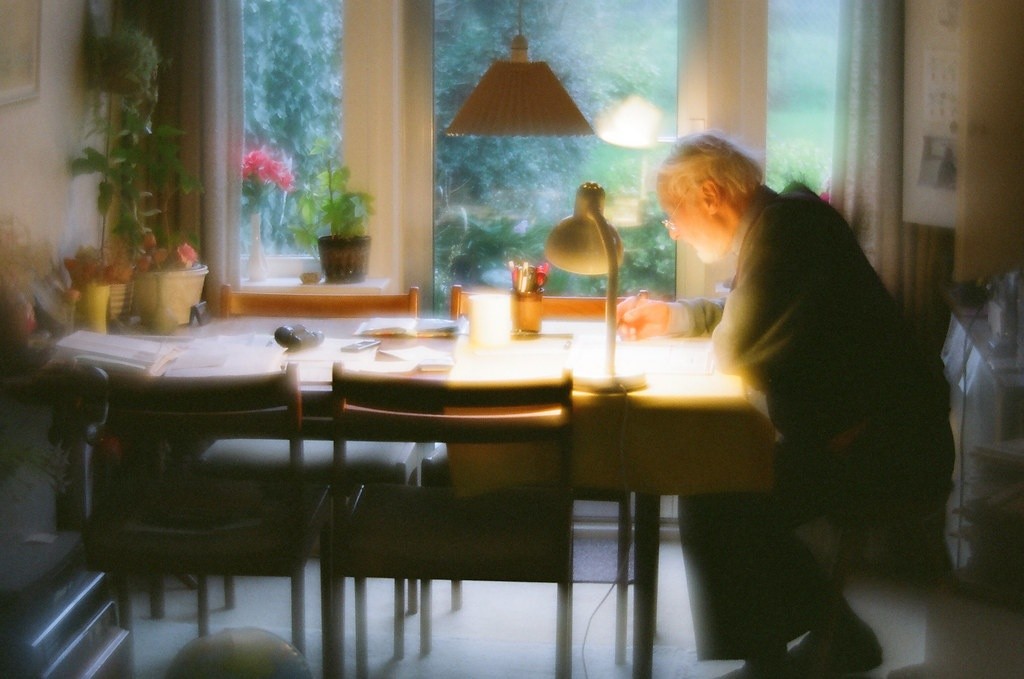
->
[511,287,544,333]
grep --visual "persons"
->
[616,133,956,679]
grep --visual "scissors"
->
[527,264,547,293]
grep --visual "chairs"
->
[449,280,627,320]
[330,363,572,679]
[80,363,302,679]
[217,282,421,321]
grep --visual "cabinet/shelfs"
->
[946,309,1024,608]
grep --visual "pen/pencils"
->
[617,290,650,332]
[506,258,550,294]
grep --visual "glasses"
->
[660,188,690,233]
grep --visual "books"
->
[353,316,469,338]
[54,330,179,377]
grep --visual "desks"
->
[39,319,778,679]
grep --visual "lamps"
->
[441,0,591,139]
[545,179,649,393]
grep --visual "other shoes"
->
[719,655,810,679]
[787,599,884,672]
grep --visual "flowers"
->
[62,28,200,280]
[242,149,294,216]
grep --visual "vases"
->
[85,283,110,336]
[105,282,128,319]
[134,266,210,334]
[251,212,266,280]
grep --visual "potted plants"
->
[295,142,374,284]
